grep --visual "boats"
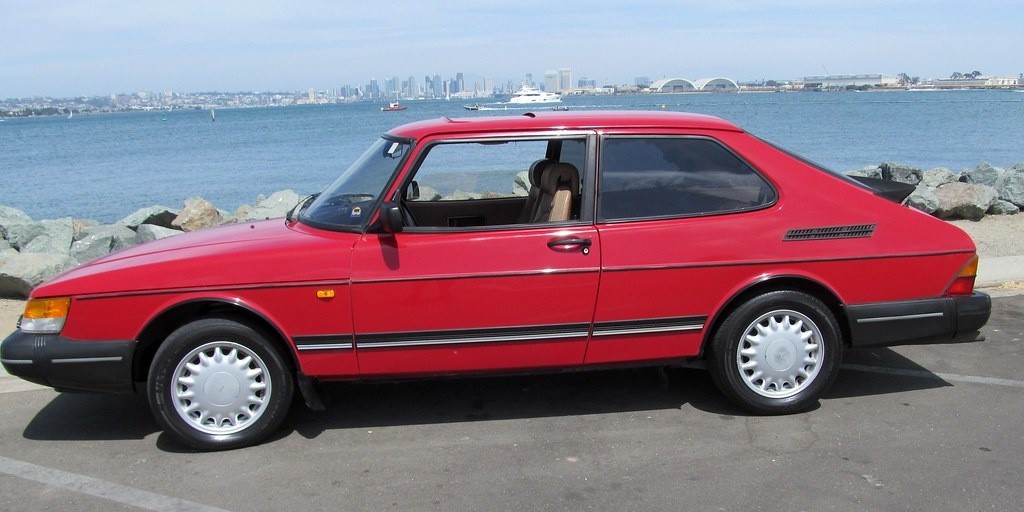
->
[464,103,478,109]
[553,105,569,112]
[381,101,407,112]
[510,82,562,103]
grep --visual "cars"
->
[0,111,992,452]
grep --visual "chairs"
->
[516,158,579,223]
